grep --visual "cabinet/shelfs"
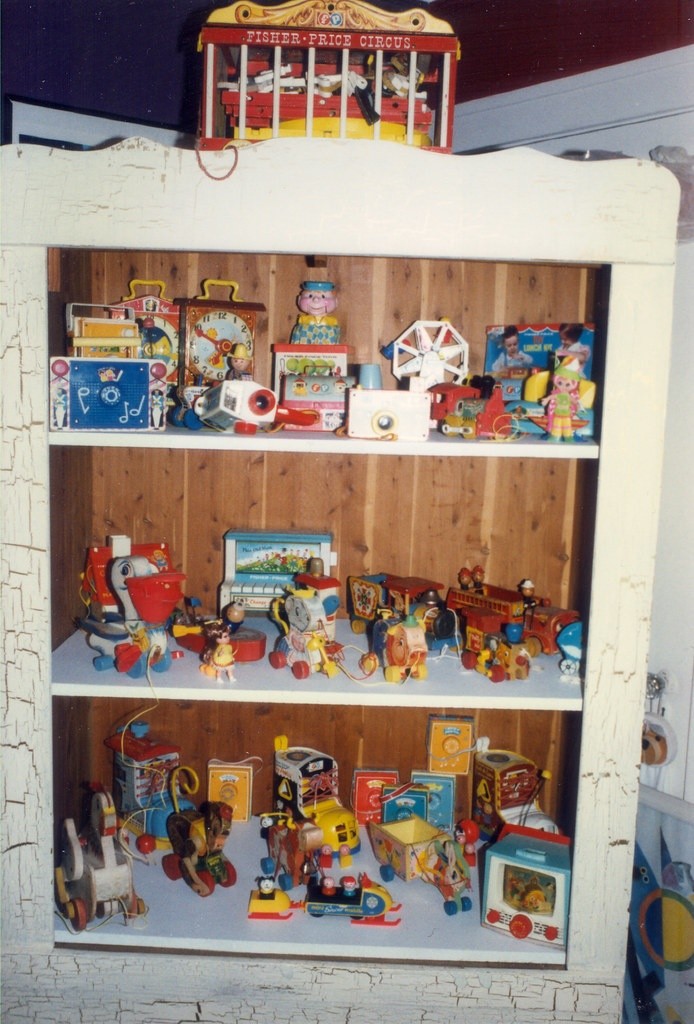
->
[48,429,601,966]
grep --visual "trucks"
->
[104,721,201,851]
[369,811,472,915]
[472,748,561,846]
[271,733,362,858]
[304,871,403,928]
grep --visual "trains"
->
[346,532,584,660]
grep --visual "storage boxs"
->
[273,342,356,404]
